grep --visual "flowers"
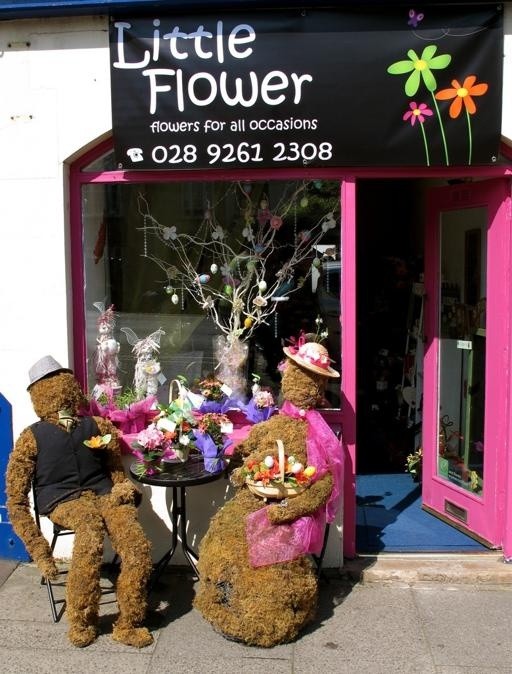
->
[384,37,490,166]
[74,373,275,477]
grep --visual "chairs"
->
[28,461,141,621]
[307,418,340,595]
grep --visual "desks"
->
[127,449,225,612]
